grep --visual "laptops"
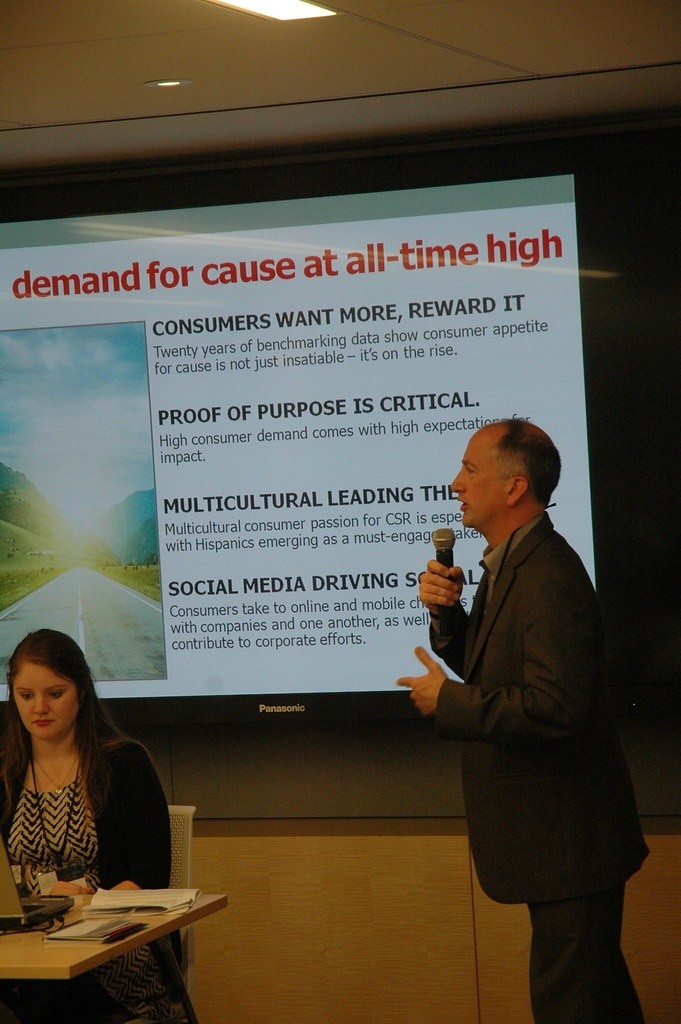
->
[0,835,74,928]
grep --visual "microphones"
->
[433,529,456,641]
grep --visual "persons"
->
[0,628,199,1024]
[395,419,652,1024]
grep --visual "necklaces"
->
[35,749,81,795]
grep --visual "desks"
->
[0,894,227,1024]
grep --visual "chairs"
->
[167,804,195,1007]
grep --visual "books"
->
[40,909,148,944]
